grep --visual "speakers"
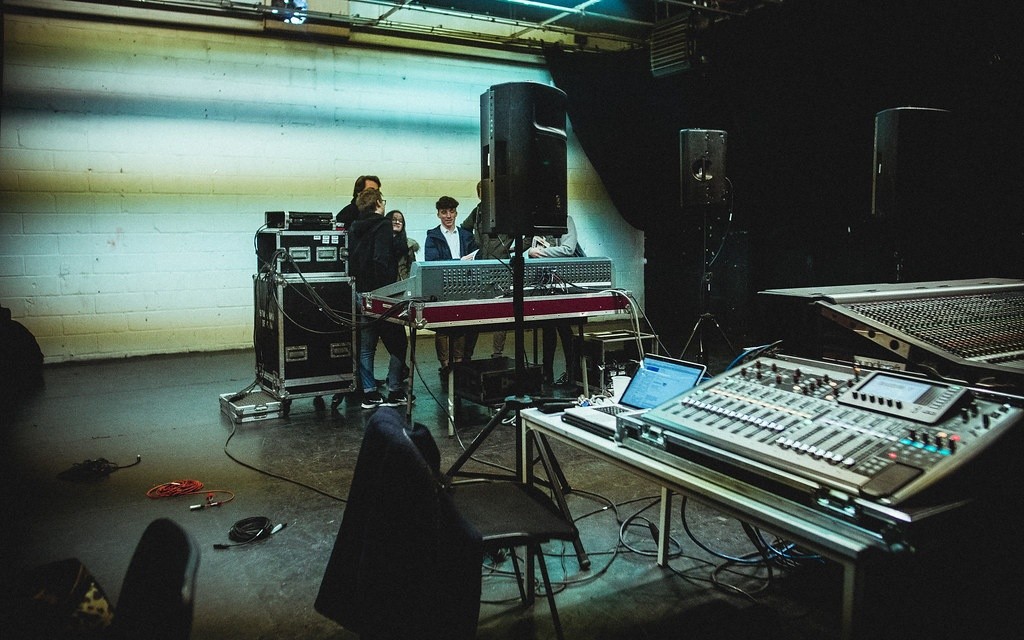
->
[679,128,730,206]
[871,107,953,215]
[481,82,568,239]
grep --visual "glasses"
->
[381,200,386,206]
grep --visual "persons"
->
[460,182,534,363]
[424,196,482,385]
[336,175,386,403]
[348,187,419,408]
[528,214,581,396]
[383,210,421,385]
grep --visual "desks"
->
[518,392,877,640]
[406,315,635,438]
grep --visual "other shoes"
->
[556,371,569,384]
[386,366,410,383]
[374,379,386,386]
[439,367,449,392]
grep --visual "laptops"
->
[563,352,707,434]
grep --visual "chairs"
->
[372,406,579,640]
[110,518,202,640]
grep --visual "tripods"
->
[447,237,591,568]
[680,205,744,377]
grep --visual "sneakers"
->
[388,391,416,406]
[361,390,398,409]
